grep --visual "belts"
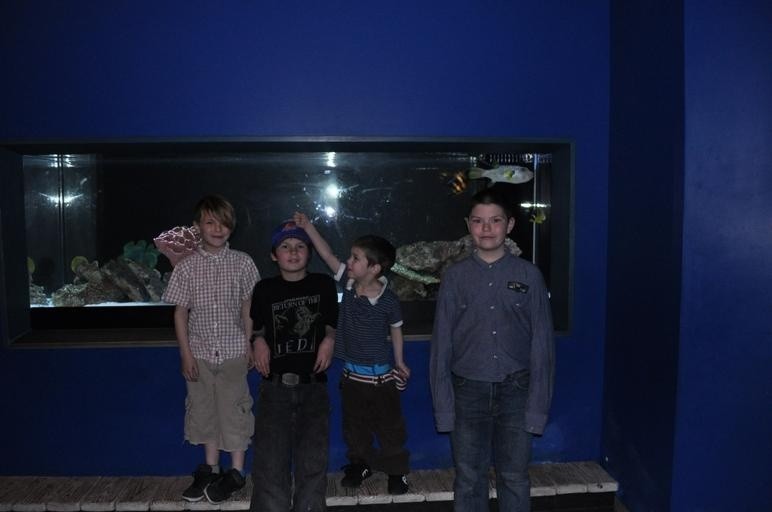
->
[342,368,408,390]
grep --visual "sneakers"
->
[387,468,410,494]
[341,462,372,487]
[203,468,246,504]
[182,463,222,501]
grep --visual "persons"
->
[291,209,412,494]
[428,191,555,512]
[249,221,338,512]
[160,191,262,504]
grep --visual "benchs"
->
[0,458,622,511]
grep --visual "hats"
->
[270,220,312,250]
